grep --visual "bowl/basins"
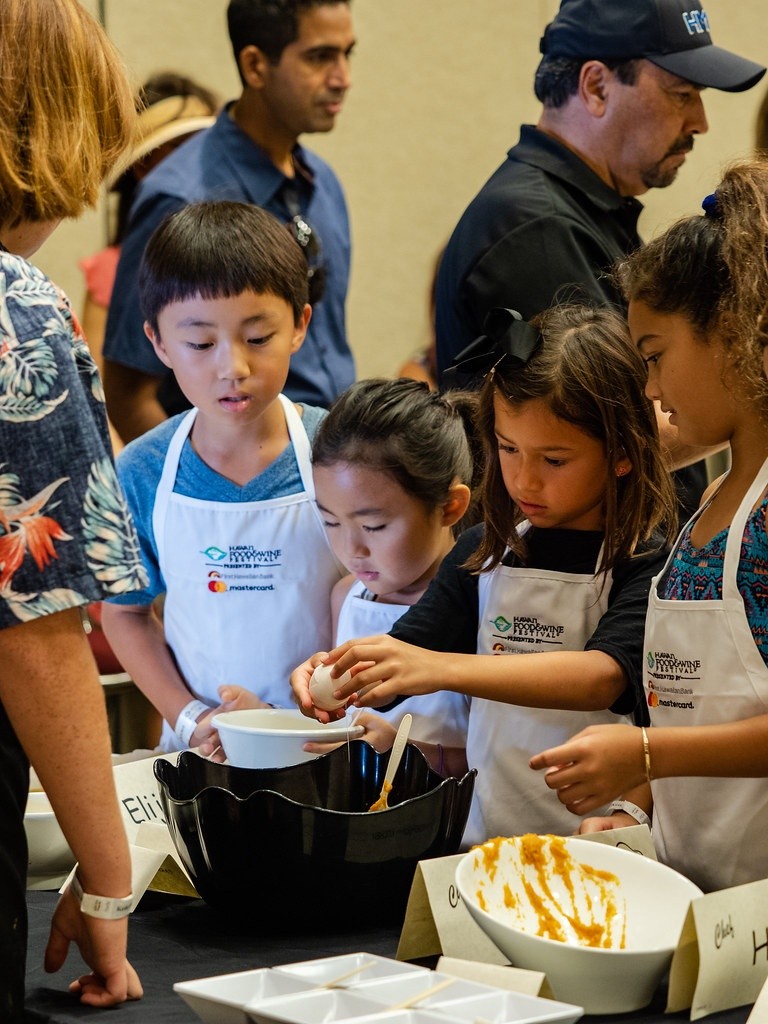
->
[152,739,478,939]
[210,708,364,769]
[455,835,704,1015]
[22,791,76,876]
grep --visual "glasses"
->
[285,215,326,305]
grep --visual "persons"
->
[526,164,768,895]
[0,1,152,1024]
[100,0,359,441]
[429,0,768,540]
[99,201,351,753]
[194,373,484,780]
[73,72,222,458]
[288,304,675,848]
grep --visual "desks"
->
[25,892,755,1024]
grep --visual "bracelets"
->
[602,799,651,831]
[174,700,210,747]
[640,725,653,781]
[435,742,444,776]
[69,868,132,920]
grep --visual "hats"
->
[106,95,219,193]
[539,0,767,93]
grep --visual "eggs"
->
[309,660,352,712]
